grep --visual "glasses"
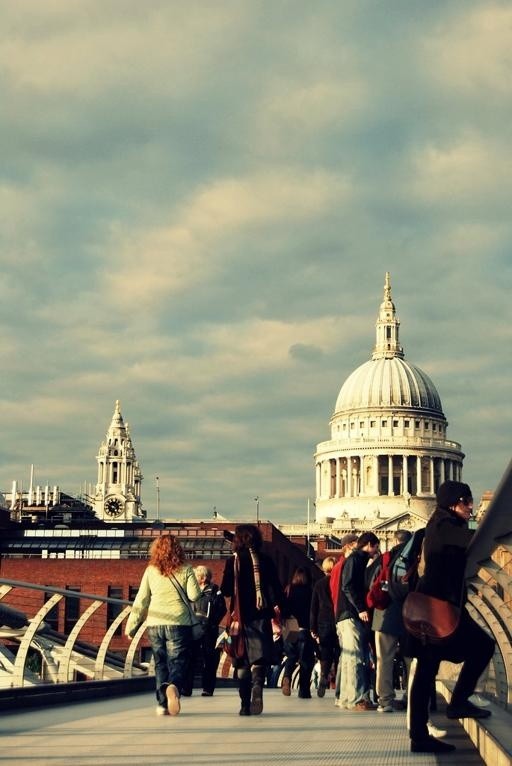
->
[455,495,474,506]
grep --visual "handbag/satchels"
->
[401,591,461,641]
[221,612,247,659]
[188,612,205,640]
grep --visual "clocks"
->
[103,497,126,518]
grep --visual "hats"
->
[435,479,471,507]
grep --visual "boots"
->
[236,677,252,716]
[250,666,266,715]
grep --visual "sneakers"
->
[409,734,457,754]
[164,683,181,715]
[351,699,376,712]
[316,677,329,698]
[156,702,168,716]
[444,704,492,722]
[377,704,394,714]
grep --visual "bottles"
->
[382,580,390,592]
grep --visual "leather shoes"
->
[282,677,291,695]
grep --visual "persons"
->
[401,481,495,750]
[124,535,201,716]
[218,524,437,716]
[181,565,227,696]
